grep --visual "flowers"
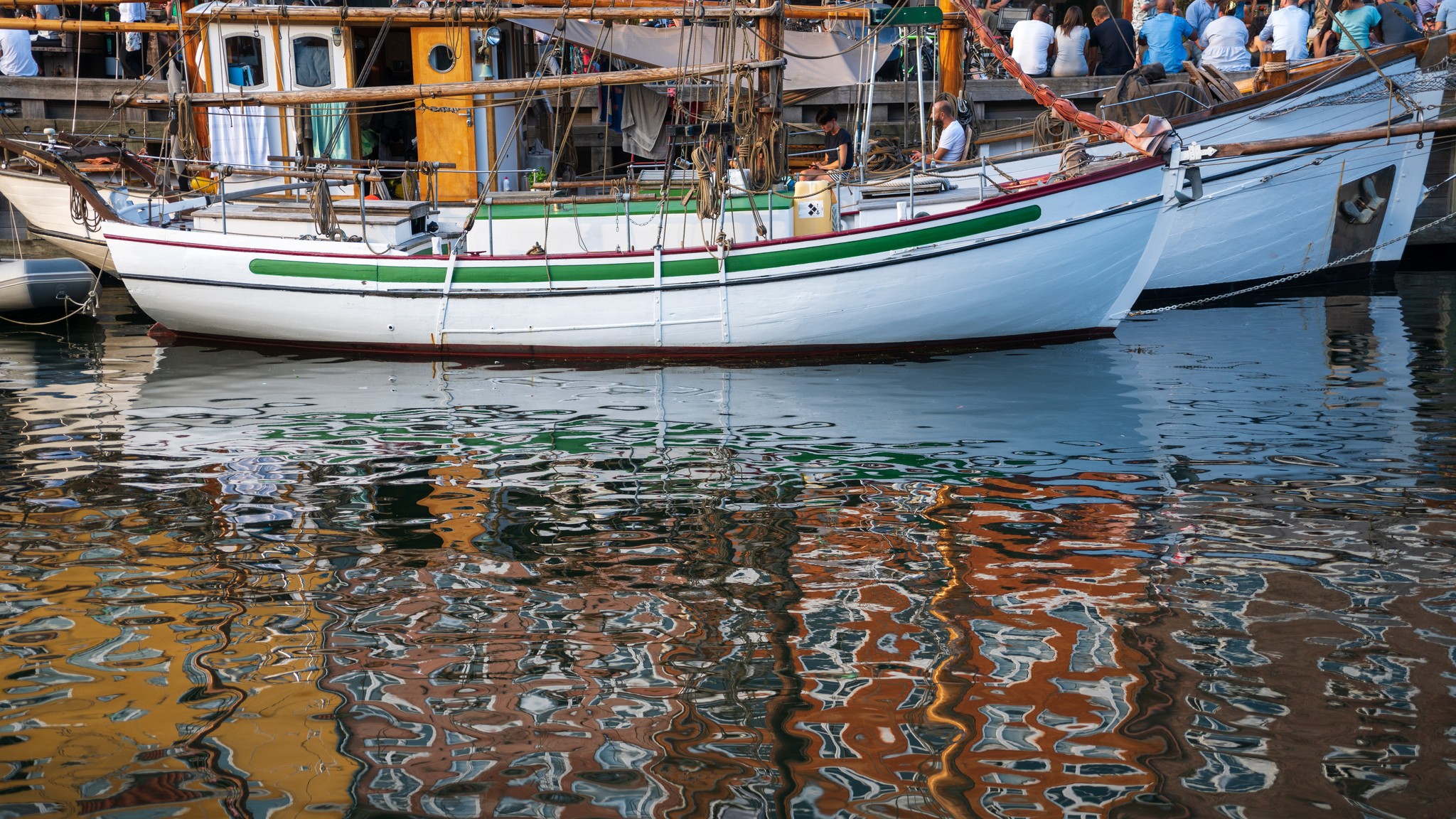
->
[1257,4,1266,11]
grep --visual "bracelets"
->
[167,18,172,20]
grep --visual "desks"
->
[145,10,170,80]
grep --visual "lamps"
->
[331,26,342,46]
[474,27,501,63]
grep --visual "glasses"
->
[1210,17,1215,22]
[1422,16,1437,23]
[1169,2,1174,8]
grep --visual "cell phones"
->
[805,164,819,167]
[906,153,919,159]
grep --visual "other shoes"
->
[172,44,183,59]
[144,76,154,80]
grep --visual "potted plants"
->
[528,167,547,191]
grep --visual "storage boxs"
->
[710,169,754,196]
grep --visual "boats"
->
[0,0,1456,372]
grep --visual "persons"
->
[1195,0,1251,72]
[1137,0,1198,73]
[1248,0,1310,65]
[1368,0,1424,49]
[1298,0,1340,59]
[1185,0,1220,69]
[1331,0,1381,53]
[1233,0,1257,27]
[962,0,1018,80]
[1131,0,1183,60]
[1050,6,1090,77]
[29,4,60,47]
[119,3,149,80]
[1414,0,1456,37]
[62,3,118,34]
[0,10,44,78]
[1087,5,1137,76]
[909,100,966,171]
[799,109,853,182]
[157,0,198,62]
[1009,4,1056,79]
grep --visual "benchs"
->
[31,17,102,79]
[73,162,163,186]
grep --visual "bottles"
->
[503,177,510,190]
